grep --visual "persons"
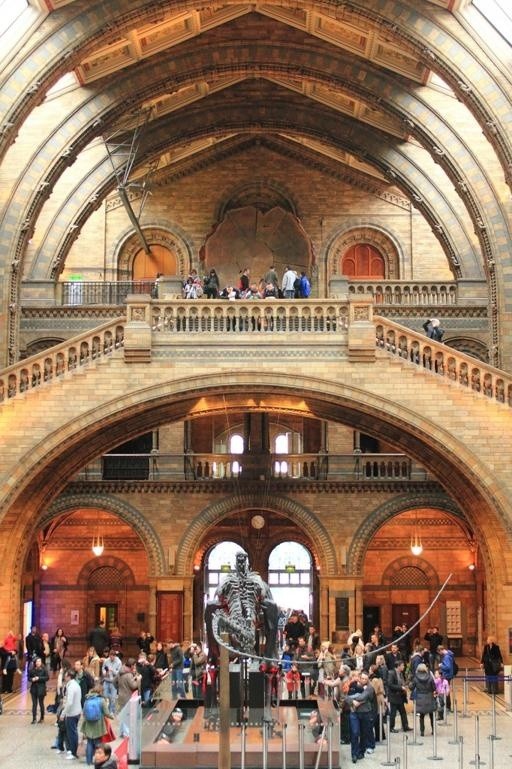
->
[1,610,460,768]
[478,634,505,694]
[415,317,446,372]
[151,263,312,330]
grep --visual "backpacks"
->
[84,694,102,720]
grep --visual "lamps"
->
[410,509,423,555]
[91,525,104,555]
